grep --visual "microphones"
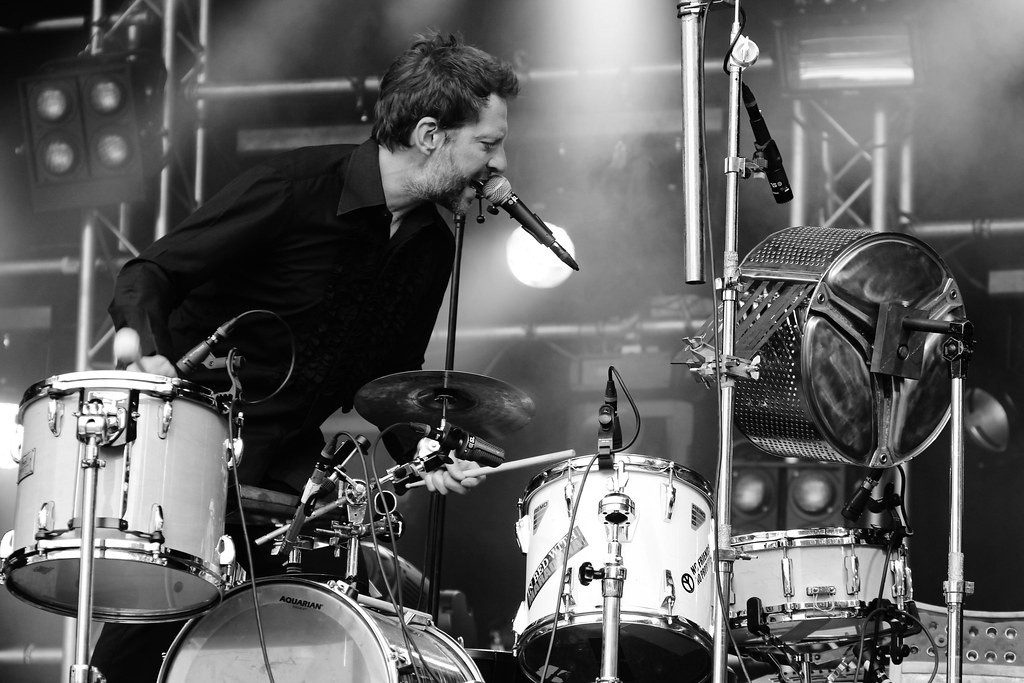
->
[408,420,507,468]
[840,469,884,524]
[742,83,794,204]
[278,438,340,557]
[604,380,622,450]
[175,319,238,378]
[482,174,580,270]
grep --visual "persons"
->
[83,29,521,683]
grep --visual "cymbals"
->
[353,368,537,440]
[726,527,925,649]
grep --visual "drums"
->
[155,574,485,683]
[510,450,720,682]
[0,369,246,625]
[728,224,970,469]
